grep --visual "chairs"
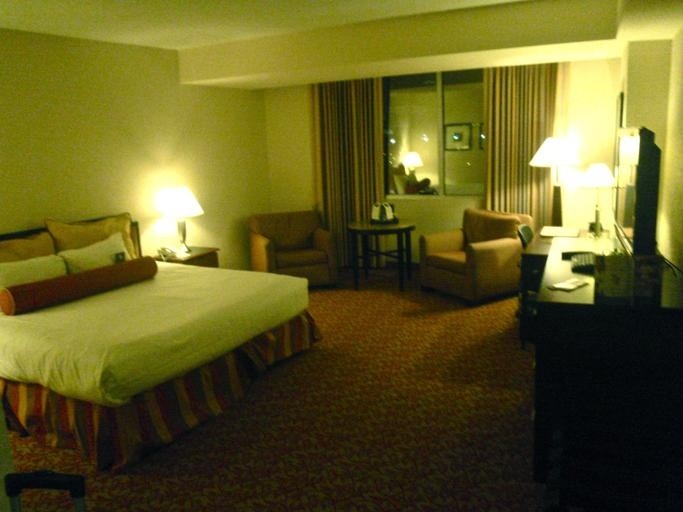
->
[246,207,340,291]
[416,207,535,304]
[514,222,537,322]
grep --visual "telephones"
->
[158,248,174,259]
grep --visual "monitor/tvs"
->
[613,127,664,264]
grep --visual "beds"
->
[1,214,312,475]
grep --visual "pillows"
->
[0,212,137,286]
[392,172,419,194]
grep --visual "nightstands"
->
[153,242,220,268]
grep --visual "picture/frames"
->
[441,122,473,152]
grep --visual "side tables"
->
[346,219,417,293]
